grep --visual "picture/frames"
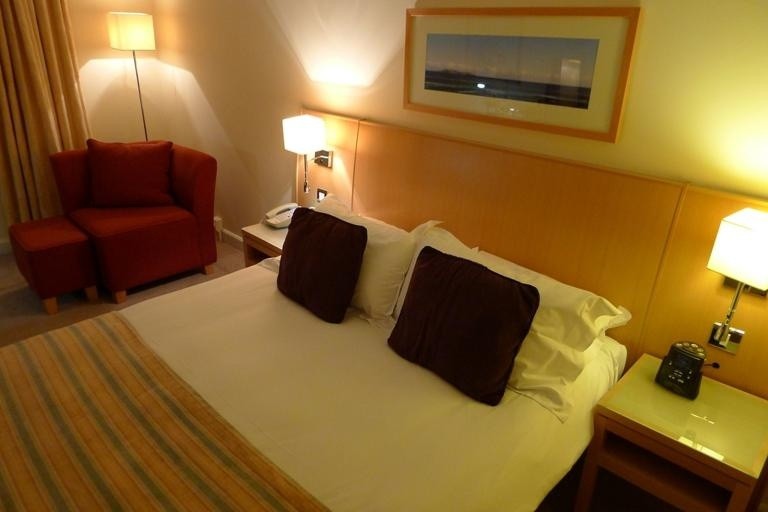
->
[399,6,643,143]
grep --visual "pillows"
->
[388,246,540,406]
[387,220,633,423]
[84,135,180,210]
[277,198,418,323]
[276,207,368,322]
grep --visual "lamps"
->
[705,203,768,358]
[105,8,159,145]
[277,113,335,196]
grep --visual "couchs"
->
[44,139,219,318]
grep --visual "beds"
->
[0,104,768,512]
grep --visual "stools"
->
[7,216,101,316]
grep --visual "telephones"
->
[264,202,302,229]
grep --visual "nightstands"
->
[239,210,290,267]
[571,350,768,510]
